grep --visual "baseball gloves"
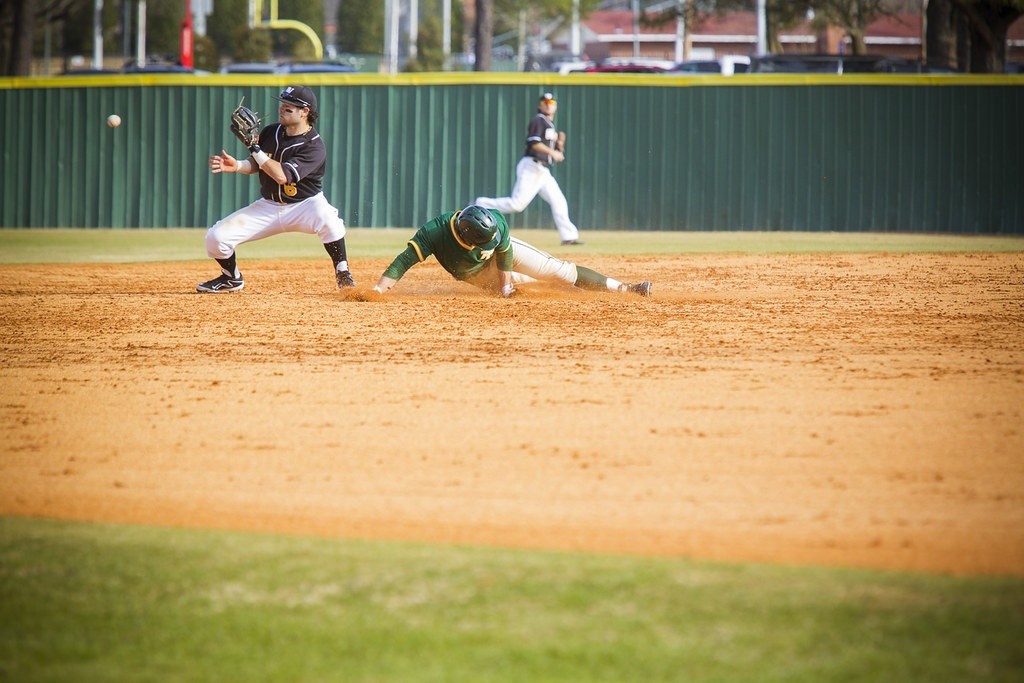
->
[229,95,272,151]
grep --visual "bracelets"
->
[500,284,515,296]
[235,159,251,175]
[250,150,271,169]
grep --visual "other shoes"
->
[562,238,585,246]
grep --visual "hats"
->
[538,92,558,102]
[271,85,317,113]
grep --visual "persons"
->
[196,86,355,293]
[372,206,652,297]
[475,93,586,244]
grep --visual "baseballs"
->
[106,114,122,128]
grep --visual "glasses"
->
[540,99,555,106]
[280,92,312,107]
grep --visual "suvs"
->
[547,53,899,75]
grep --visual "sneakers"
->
[196,272,244,292]
[336,271,355,289]
[618,282,651,298]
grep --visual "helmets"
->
[458,205,497,244]
